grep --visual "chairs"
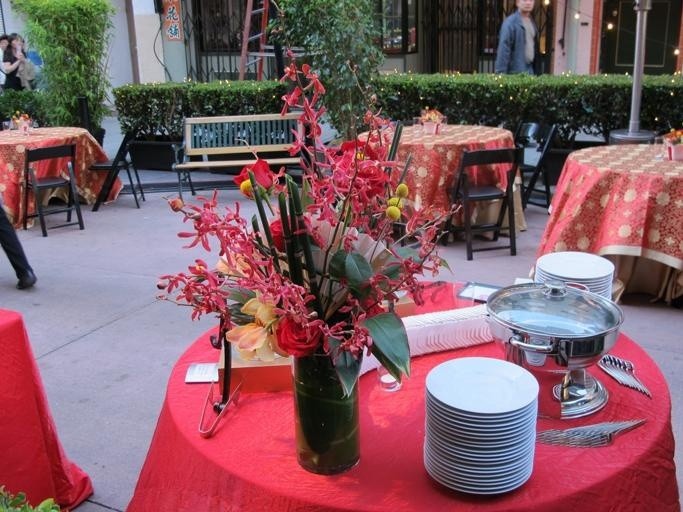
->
[447,146,526,262]
[513,119,558,215]
[79,127,149,215]
[20,142,87,237]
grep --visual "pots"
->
[484,277,624,420]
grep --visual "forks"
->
[536,419,647,449]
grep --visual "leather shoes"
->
[16,270,37,289]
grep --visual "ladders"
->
[238,1,268,80]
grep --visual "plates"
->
[422,356,538,495]
[535,251,616,306]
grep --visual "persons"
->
[0,198,36,291]
[3,33,35,91]
[495,0,541,77]
[0,34,10,94]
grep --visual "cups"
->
[377,359,403,391]
[412,116,421,136]
[423,122,436,142]
[3,121,10,137]
[654,136,664,159]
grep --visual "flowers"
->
[419,108,446,124]
[152,48,508,396]
[12,108,34,125]
[664,126,683,143]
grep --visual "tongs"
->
[197,379,243,439]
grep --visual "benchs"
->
[171,109,307,203]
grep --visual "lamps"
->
[569,1,585,29]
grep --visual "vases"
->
[666,141,683,161]
[287,322,366,477]
[17,125,29,136]
[424,122,438,135]
